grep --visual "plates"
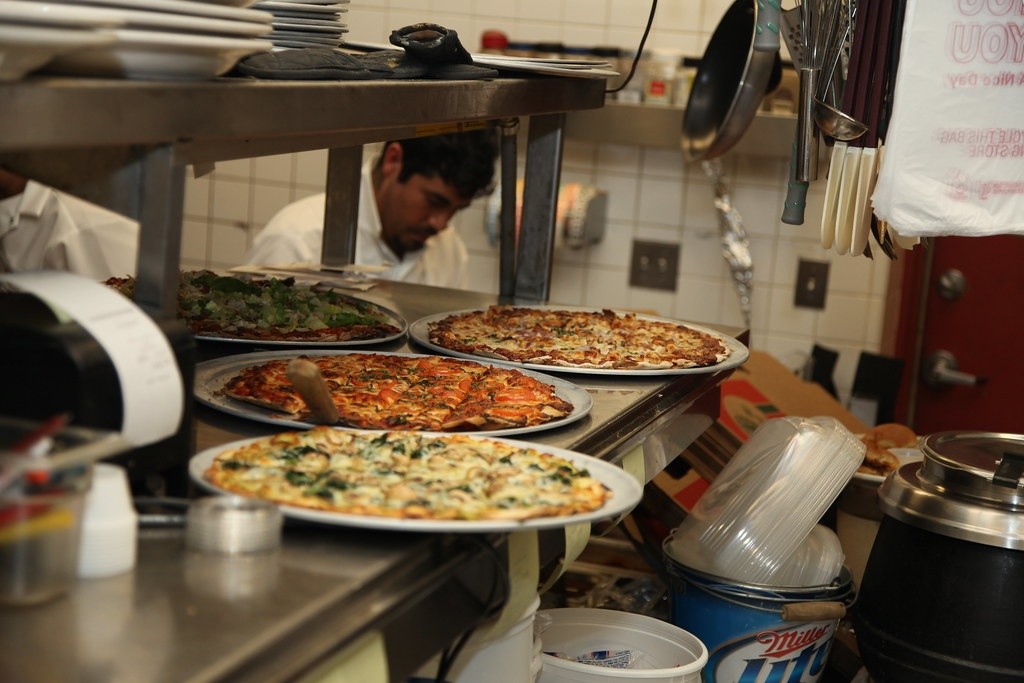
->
[1,0,358,93]
[99,271,410,345]
[189,430,641,528]
[406,301,751,373]
[188,350,593,443]
[844,427,928,488]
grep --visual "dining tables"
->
[23,71,606,326]
[0,264,737,683]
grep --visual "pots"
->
[873,429,1024,683]
[678,0,782,165]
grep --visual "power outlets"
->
[626,240,684,294]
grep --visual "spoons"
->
[775,0,930,267]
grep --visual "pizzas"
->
[101,269,729,521]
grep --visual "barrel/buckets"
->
[654,534,857,683]
[532,607,710,682]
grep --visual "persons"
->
[221,132,509,290]
